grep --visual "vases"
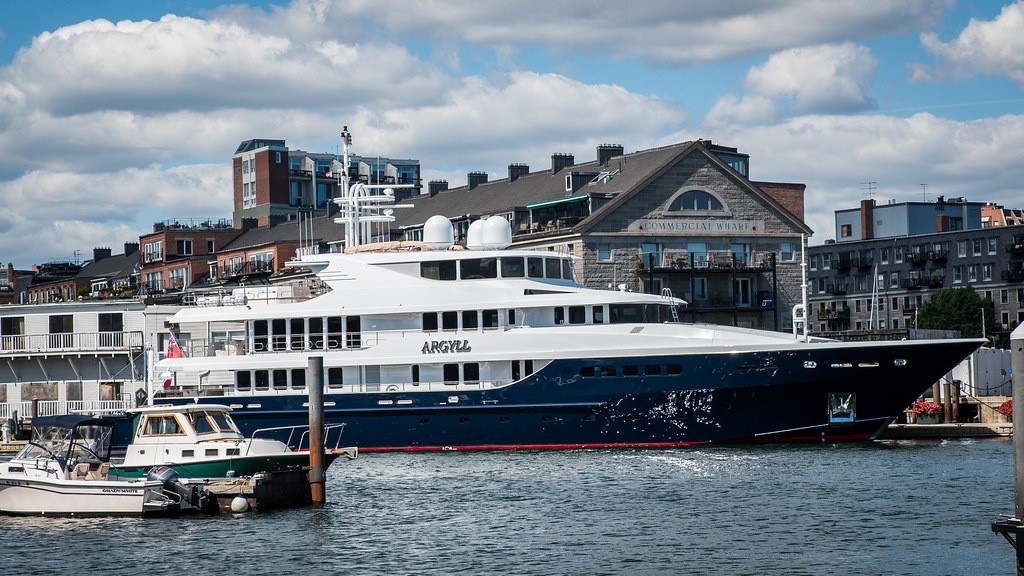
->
[1008,417,1012,422]
[917,414,941,423]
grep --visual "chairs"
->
[72,462,112,480]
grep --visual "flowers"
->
[998,400,1013,416]
[913,402,942,416]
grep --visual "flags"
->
[167,336,183,358]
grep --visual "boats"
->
[0,119,995,454]
[0,350,359,519]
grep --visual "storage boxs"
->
[762,299,773,306]
